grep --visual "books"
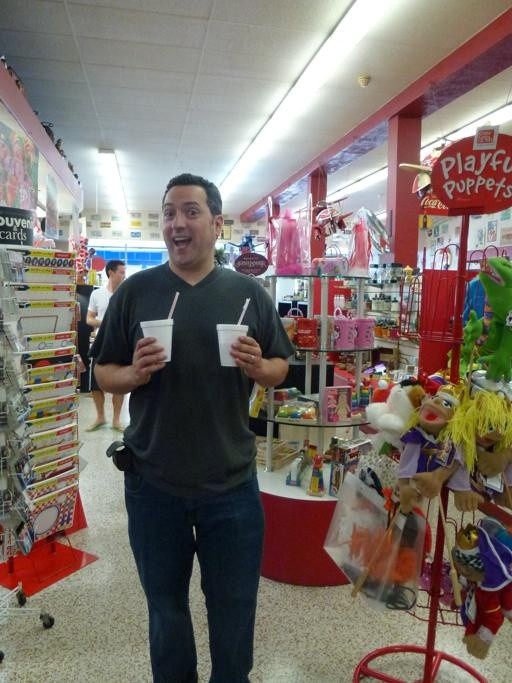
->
[0,243,83,548]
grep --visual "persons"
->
[87,171,298,681]
[83,260,127,433]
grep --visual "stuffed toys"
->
[359,372,511,660]
[444,252,512,384]
[359,372,511,659]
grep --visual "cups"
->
[139,318,177,363]
[280,316,374,349]
[215,323,249,367]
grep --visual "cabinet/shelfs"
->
[253,273,375,588]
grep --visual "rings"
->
[250,354,256,363]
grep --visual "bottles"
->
[275,210,301,276]
[308,456,326,496]
[372,292,400,311]
[368,262,403,282]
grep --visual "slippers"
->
[110,425,126,433]
[84,419,109,432]
